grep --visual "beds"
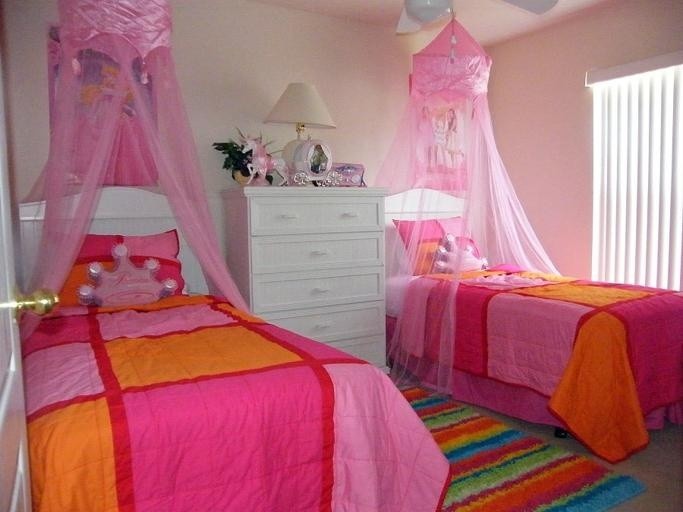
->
[10,184,449,511]
[379,189,681,464]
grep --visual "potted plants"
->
[214,128,283,184]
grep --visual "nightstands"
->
[208,173,398,380]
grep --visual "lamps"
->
[263,81,340,183]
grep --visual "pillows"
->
[392,214,483,272]
[41,229,188,311]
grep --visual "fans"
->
[395,0,558,34]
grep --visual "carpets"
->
[393,387,646,511]
